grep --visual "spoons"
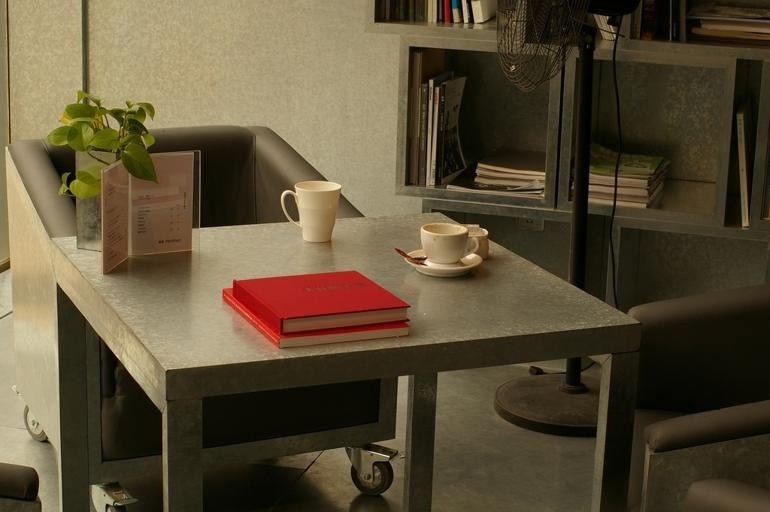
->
[393,247,428,265]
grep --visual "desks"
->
[43,209,633,512]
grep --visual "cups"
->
[459,228,489,262]
[280,178,342,244]
[420,222,480,265]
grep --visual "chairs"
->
[5,126,411,512]
[625,298,767,510]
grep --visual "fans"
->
[493,0,626,437]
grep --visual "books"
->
[222,270,411,349]
[588,143,672,208]
[737,97,756,228]
[379,1,498,24]
[594,1,769,44]
[409,51,546,199]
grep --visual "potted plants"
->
[45,96,159,250]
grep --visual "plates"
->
[404,249,484,278]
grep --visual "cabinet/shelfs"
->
[362,1,767,293]
